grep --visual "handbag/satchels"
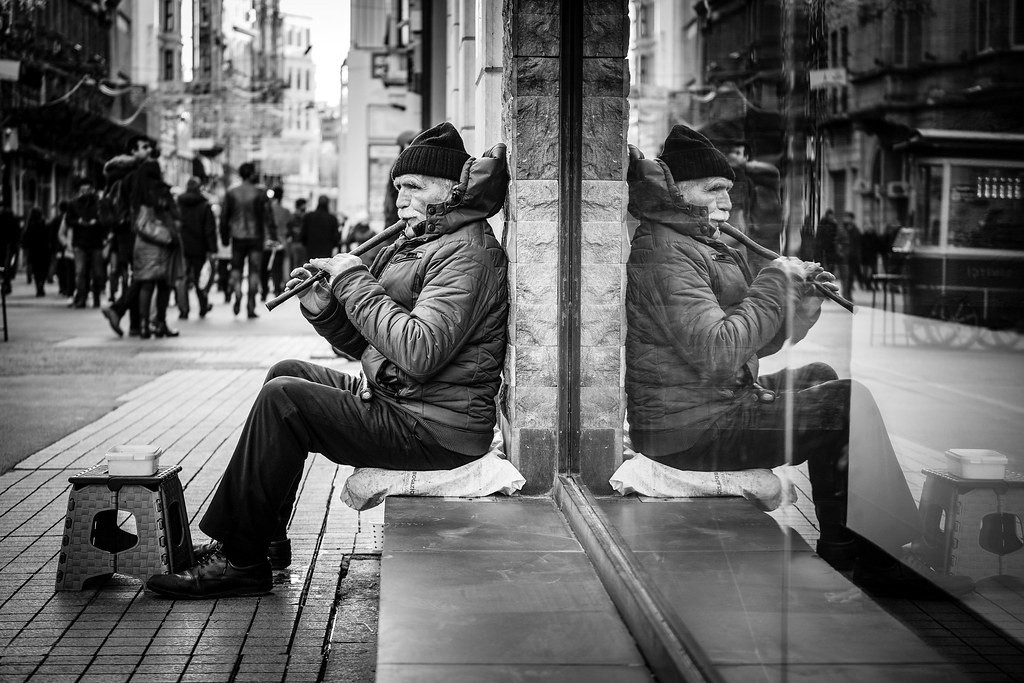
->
[134,206,173,247]
[198,259,212,290]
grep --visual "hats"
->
[660,123,735,185]
[390,121,472,185]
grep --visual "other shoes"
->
[248,309,258,318]
[129,330,141,336]
[199,304,213,316]
[155,322,178,338]
[179,312,189,318]
[812,535,934,588]
[234,298,241,314]
[102,308,123,337]
[141,327,152,338]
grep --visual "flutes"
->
[719,222,862,315]
[263,219,408,312]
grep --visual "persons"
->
[626,123,981,598]
[0,132,418,340]
[147,124,509,599]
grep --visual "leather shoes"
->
[194,539,292,569]
[145,549,274,598]
